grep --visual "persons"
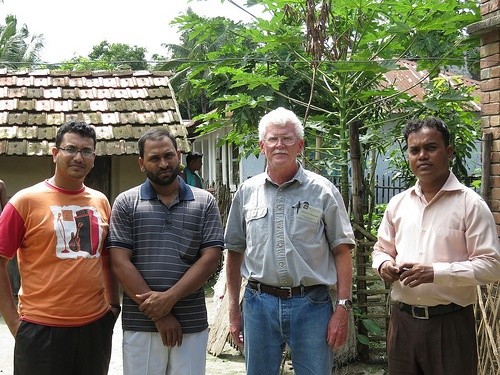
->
[104,129,224,375]
[224,118,356,375]
[371,120,500,375]
[0,121,121,375]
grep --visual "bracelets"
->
[109,304,121,311]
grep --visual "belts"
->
[399,302,459,320]
[248,280,320,299]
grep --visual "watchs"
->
[336,299,352,311]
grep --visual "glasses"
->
[58,146,95,157]
[264,136,297,145]
